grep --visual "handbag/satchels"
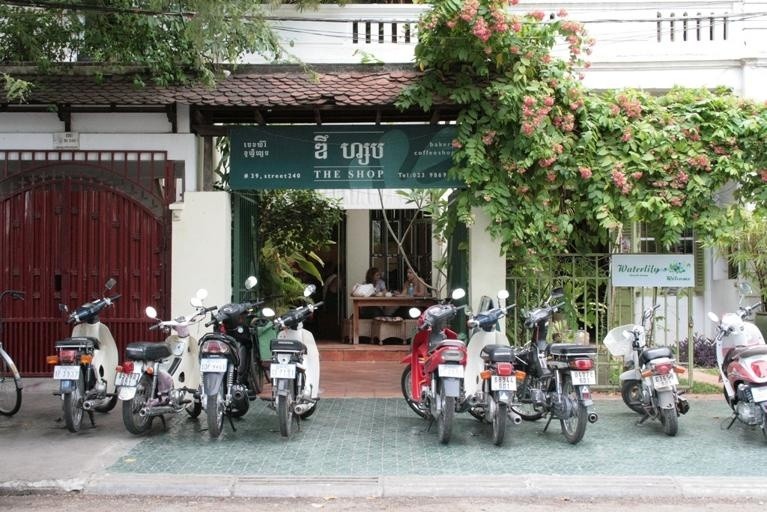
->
[350,282,376,298]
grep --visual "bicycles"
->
[0,289,24,417]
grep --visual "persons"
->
[391,267,428,297]
[323,268,343,306]
[363,267,387,317]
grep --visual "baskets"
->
[603,324,645,356]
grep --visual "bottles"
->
[408,282,413,297]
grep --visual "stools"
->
[342,317,420,345]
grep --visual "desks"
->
[349,296,441,345]
